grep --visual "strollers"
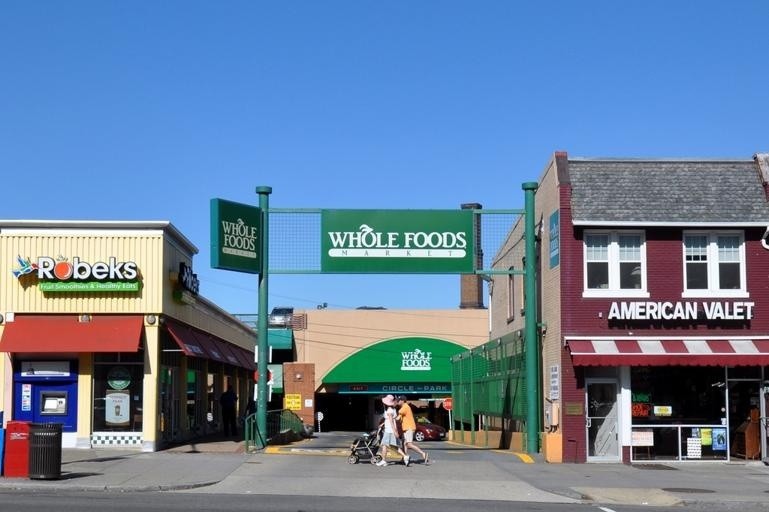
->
[346,423,385,467]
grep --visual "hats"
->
[382,394,395,406]
[397,395,406,400]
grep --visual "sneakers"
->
[422,451,428,464]
[395,455,410,466]
[376,460,388,467]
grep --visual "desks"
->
[632,427,655,460]
[133,414,142,431]
[649,449,649,451]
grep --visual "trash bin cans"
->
[27,422,64,481]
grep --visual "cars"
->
[378,415,446,442]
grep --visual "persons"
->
[376,395,410,467]
[395,395,428,464]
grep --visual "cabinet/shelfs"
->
[730,408,761,460]
